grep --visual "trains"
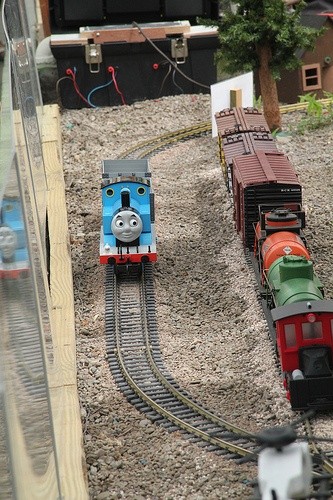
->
[215,105,332,415]
[0,195,30,280]
[99,160,156,280]
